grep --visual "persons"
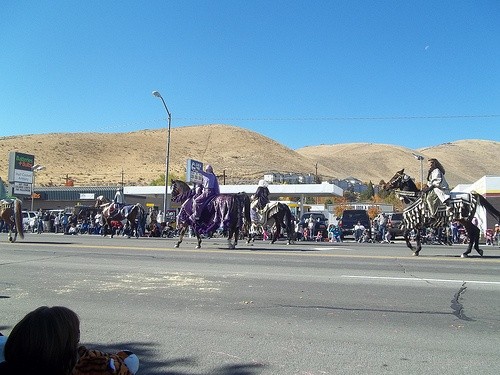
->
[188,165,220,220]
[0,306,80,375]
[111,188,124,214]
[249,179,270,227]
[423,158,452,223]
[23,206,500,246]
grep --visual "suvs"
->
[299,212,329,238]
[22,211,39,230]
[381,212,410,239]
[338,209,371,242]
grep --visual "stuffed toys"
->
[70,346,140,375]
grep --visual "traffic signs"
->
[186,159,203,185]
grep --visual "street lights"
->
[152,91,172,222]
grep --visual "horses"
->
[383,168,500,259]
[95,195,146,239]
[239,192,295,245]
[170,178,251,249]
[0,198,24,243]
